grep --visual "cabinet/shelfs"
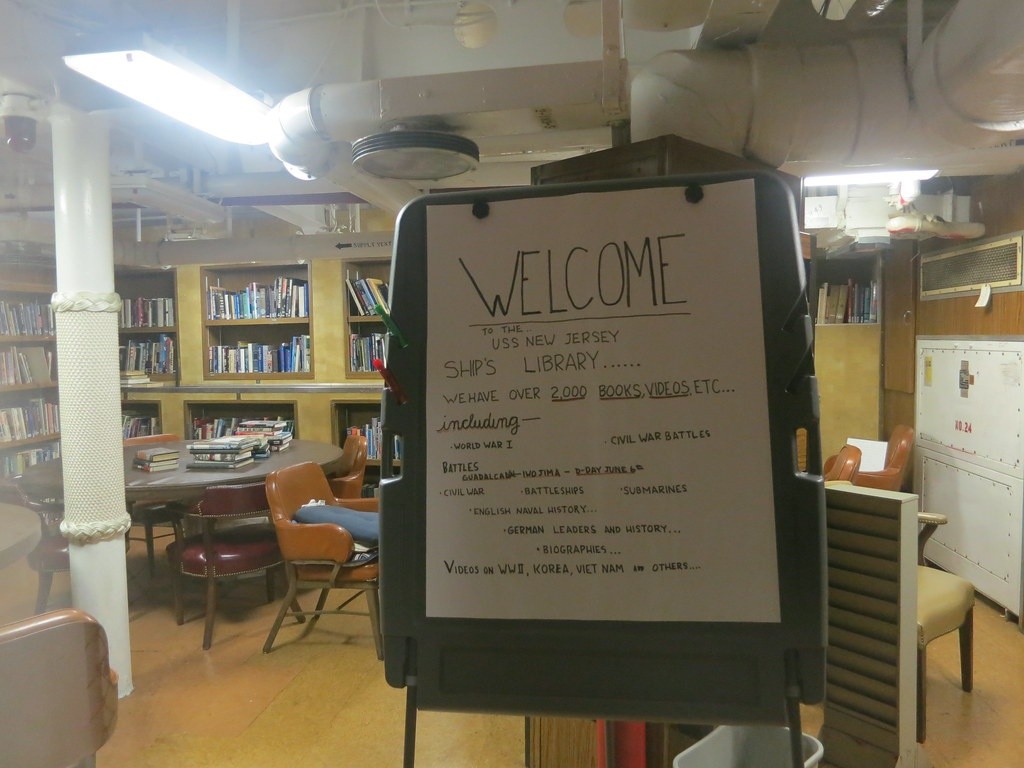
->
[0,254,404,475]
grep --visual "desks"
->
[26,440,344,624]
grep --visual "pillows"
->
[292,505,382,546]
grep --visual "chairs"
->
[4,475,74,618]
[261,461,383,663]
[820,423,976,744]
[166,479,305,651]
[321,432,369,496]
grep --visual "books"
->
[186,416,295,468]
[122,414,161,439]
[133,448,181,472]
[207,277,310,373]
[347,418,400,498]
[118,297,177,387]
[817,277,877,324]
[0,301,62,478]
[346,278,390,372]
